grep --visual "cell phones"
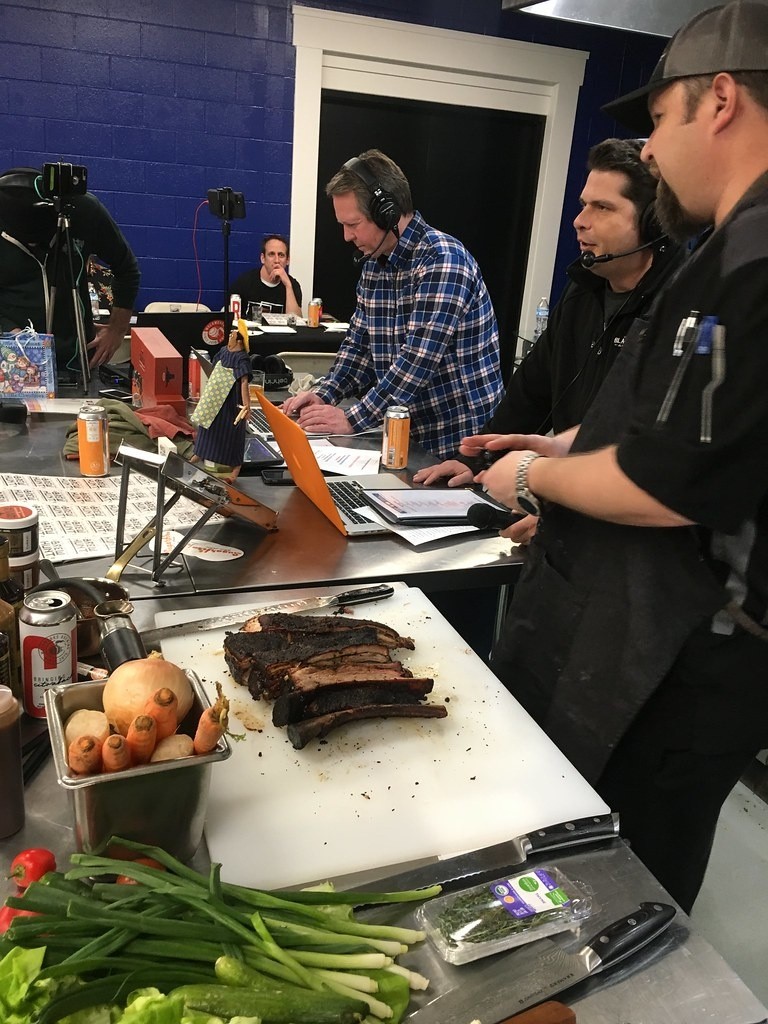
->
[41,163,87,195]
[207,189,246,219]
[259,469,296,486]
[98,388,132,402]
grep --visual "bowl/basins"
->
[23,576,131,658]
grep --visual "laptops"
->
[256,391,413,536]
[190,346,332,440]
[99,311,235,388]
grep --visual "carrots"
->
[67,688,229,775]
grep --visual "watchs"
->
[516,453,556,517]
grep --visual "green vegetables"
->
[434,887,562,945]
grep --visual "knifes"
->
[399,901,678,1024]
[138,584,394,645]
[335,812,622,904]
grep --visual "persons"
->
[0,168,140,371]
[412,137,669,547]
[227,236,302,317]
[458,0,768,916]
[277,149,506,462]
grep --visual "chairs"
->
[96,335,131,367]
[146,301,209,313]
[278,350,339,380]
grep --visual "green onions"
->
[0,836,442,1024]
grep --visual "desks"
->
[89,313,352,384]
[0,396,543,661]
[0,580,768,1023]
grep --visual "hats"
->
[599,0,768,120]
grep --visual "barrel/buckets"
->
[41,667,233,884]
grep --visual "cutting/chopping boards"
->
[153,586,613,893]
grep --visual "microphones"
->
[358,218,392,265]
[468,503,526,531]
[580,238,664,268]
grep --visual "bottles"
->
[535,297,549,340]
[0,532,28,842]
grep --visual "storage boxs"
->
[0,332,58,399]
[44,667,232,869]
[132,381,187,418]
[130,327,183,394]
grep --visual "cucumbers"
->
[161,982,365,1024]
[214,955,371,1022]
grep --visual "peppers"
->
[3,847,56,888]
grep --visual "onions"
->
[102,651,195,738]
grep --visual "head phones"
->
[248,354,295,390]
[639,138,676,251]
[345,157,401,239]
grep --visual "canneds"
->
[77,405,110,478]
[18,590,78,720]
[381,405,410,470]
[230,294,241,327]
[308,298,322,327]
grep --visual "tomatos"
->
[0,892,47,938]
[114,857,166,885]
